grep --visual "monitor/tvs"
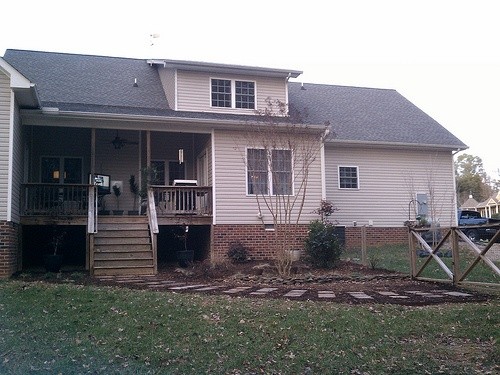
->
[88,173,110,190]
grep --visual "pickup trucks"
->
[457,210,500,243]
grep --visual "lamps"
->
[113,130,122,149]
